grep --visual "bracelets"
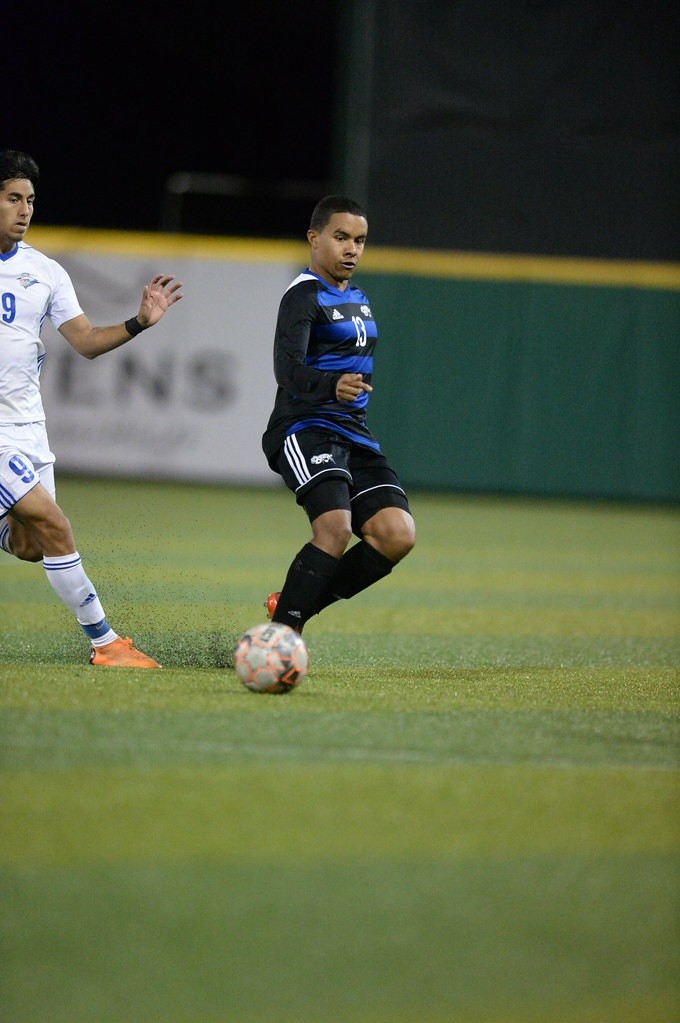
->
[124,315,144,335]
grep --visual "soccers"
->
[232,620,311,696]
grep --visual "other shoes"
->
[265,591,300,636]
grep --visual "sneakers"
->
[90,636,162,667]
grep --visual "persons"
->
[261,192,418,639]
[0,148,184,670]
